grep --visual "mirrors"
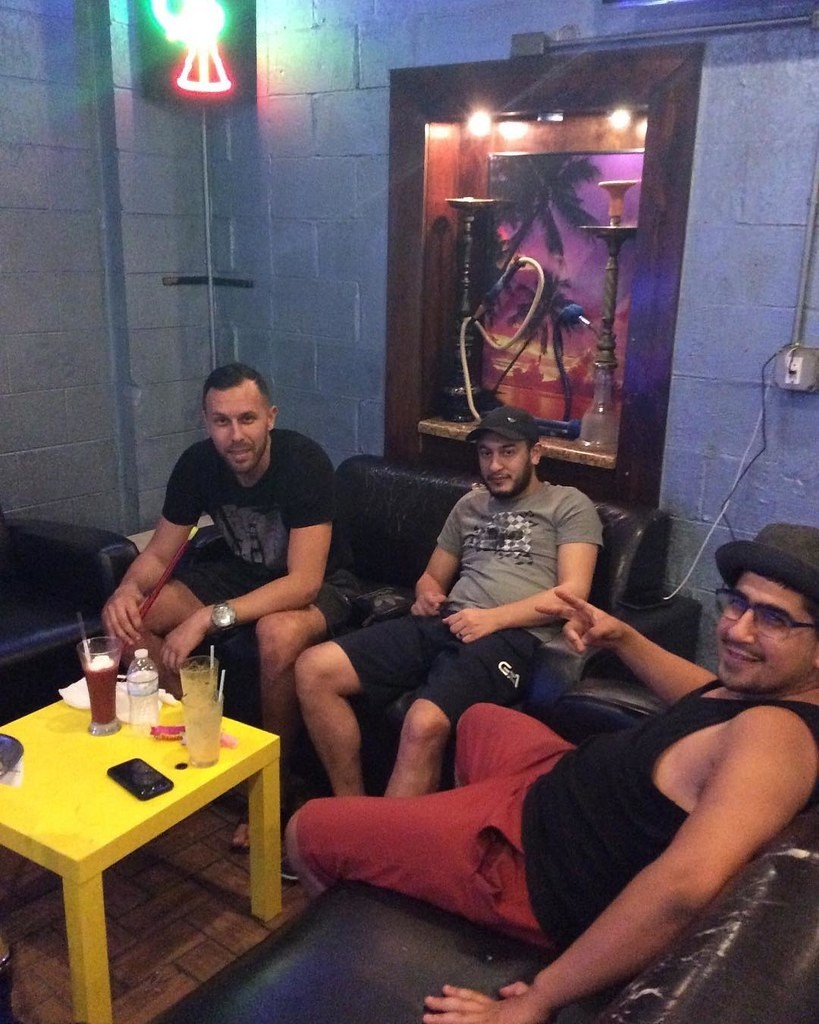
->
[386,42,704,505]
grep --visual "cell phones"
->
[107,758,174,802]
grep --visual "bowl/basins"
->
[0,734,24,780]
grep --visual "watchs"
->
[209,602,238,629]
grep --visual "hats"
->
[466,406,539,443]
[715,521,819,605]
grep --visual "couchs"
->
[0,510,139,725]
[169,454,703,807]
[150,680,819,1024]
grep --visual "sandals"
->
[231,812,249,854]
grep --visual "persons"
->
[283,524,819,1024]
[280,407,606,882]
[98,363,357,854]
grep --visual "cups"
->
[179,655,223,764]
[76,638,125,735]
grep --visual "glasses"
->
[716,587,818,640]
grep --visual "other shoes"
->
[279,857,299,881]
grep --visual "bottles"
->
[128,648,159,731]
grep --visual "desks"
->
[0,699,282,1024]
